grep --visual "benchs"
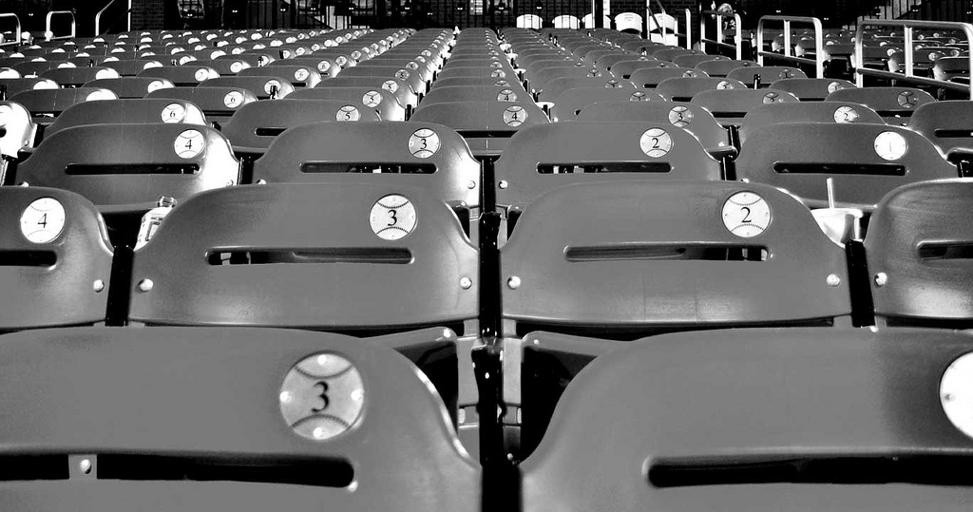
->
[1,22,970,512]
